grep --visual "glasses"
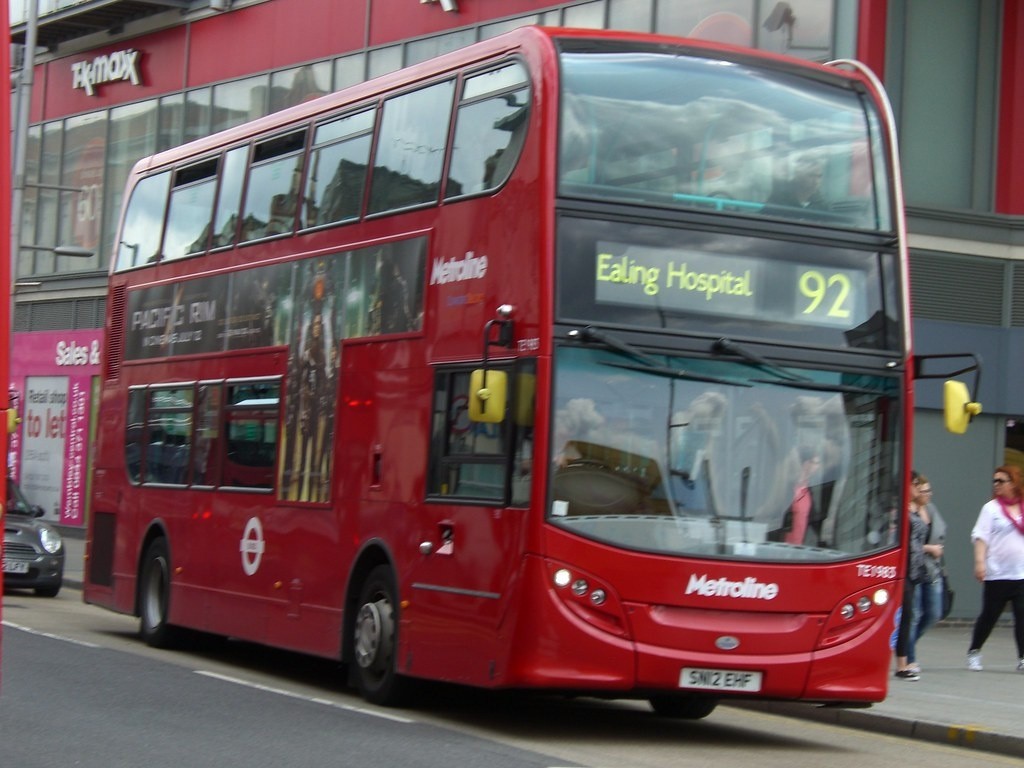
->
[911,482,919,486]
[992,477,1012,485]
[920,487,933,493]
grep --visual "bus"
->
[144,399,278,487]
[82,23,986,719]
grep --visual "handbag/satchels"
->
[941,576,955,621]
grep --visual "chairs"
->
[124,427,278,489]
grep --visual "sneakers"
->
[896,670,920,681]
[1018,656,1024,672]
[965,648,982,672]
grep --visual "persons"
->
[286,311,337,505]
[966,466,1024,671]
[895,471,928,681]
[556,390,846,546]
[907,475,946,673]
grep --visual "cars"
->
[2,477,65,596]
